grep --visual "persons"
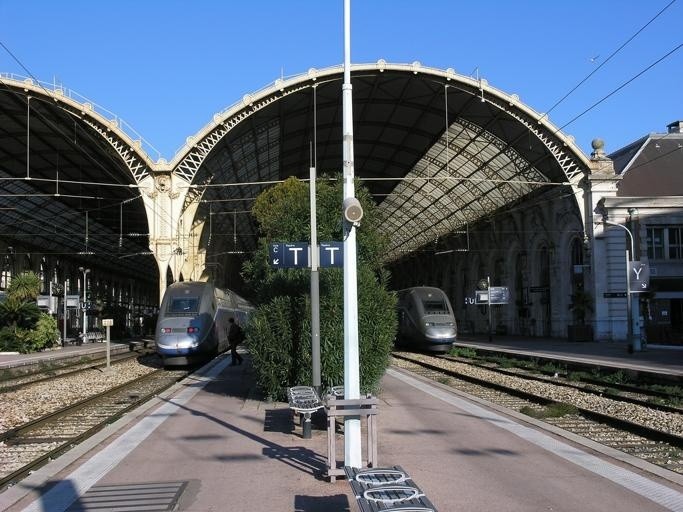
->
[227,317,245,367]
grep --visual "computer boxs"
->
[342,197,363,223]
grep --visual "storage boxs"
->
[343,464,438,512]
[286,385,325,439]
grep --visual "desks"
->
[594,215,634,262]
[78,266,92,342]
